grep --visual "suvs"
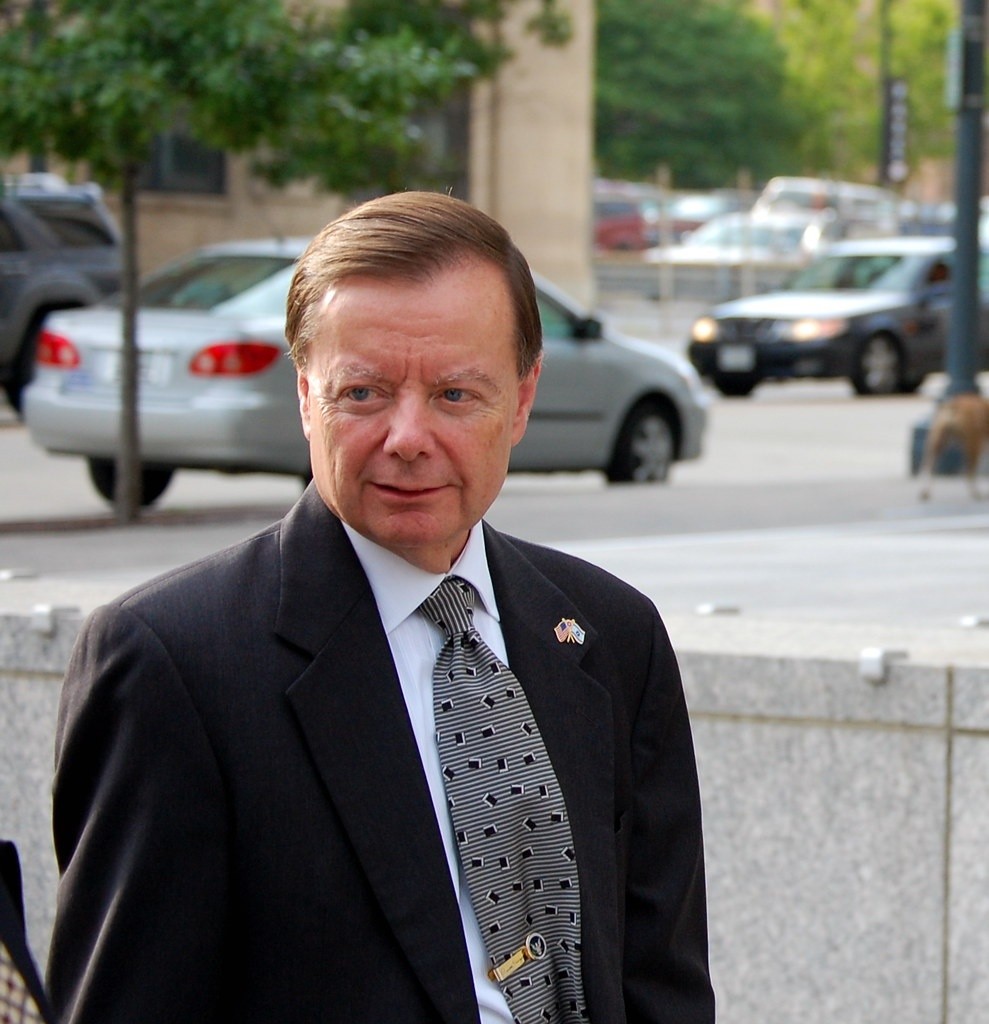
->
[0,174,126,417]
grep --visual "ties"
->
[416,574,589,1024]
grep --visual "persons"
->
[43,192,718,1024]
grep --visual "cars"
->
[689,236,989,397]
[27,240,708,518]
[595,173,989,266]
